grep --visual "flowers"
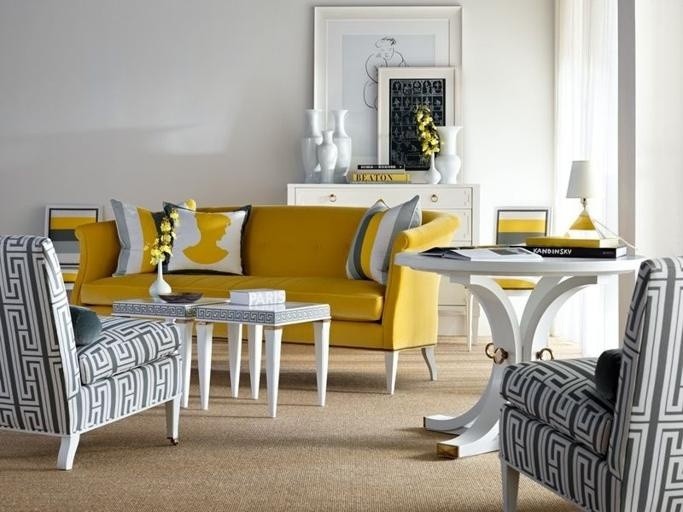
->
[143,209,180,266]
[413,105,441,156]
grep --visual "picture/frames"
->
[378,66,461,182]
[313,5,463,182]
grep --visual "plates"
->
[156,291,204,303]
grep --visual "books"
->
[350,163,430,184]
[419,236,628,263]
[228,287,287,305]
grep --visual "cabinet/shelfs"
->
[287,183,480,352]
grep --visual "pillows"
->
[345,194,423,284]
[109,197,197,277]
[161,200,252,276]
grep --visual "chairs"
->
[468,206,554,352]
[0,236,183,471]
[44,204,104,293]
[499,255,683,512]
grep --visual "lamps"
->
[565,159,605,241]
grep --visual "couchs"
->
[69,204,461,394]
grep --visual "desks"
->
[394,252,644,458]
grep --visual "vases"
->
[316,130,338,183]
[435,124,462,184]
[327,108,353,183]
[424,152,442,184]
[148,261,173,302]
[299,109,323,183]
